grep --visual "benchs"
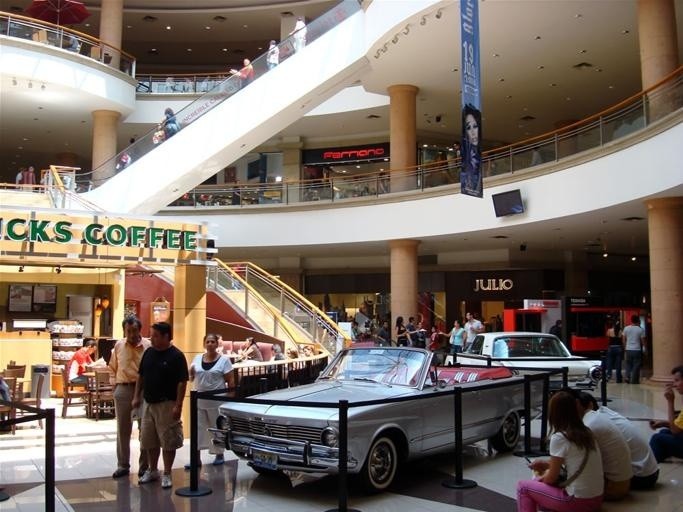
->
[600,454,682,512]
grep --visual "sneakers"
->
[161,473,173,487]
[214,456,224,465]
[113,468,128,478]
[184,463,190,467]
[137,467,160,482]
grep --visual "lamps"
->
[94,267,109,317]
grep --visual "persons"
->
[182,333,237,469]
[461,102,483,198]
[215,333,224,356]
[115,151,130,172]
[571,391,634,502]
[14,166,25,189]
[266,40,279,71]
[62,32,78,51]
[648,365,682,463]
[152,107,179,145]
[266,341,317,380]
[238,336,263,360]
[129,321,190,488]
[548,319,563,341]
[22,166,36,192]
[286,17,306,54]
[231,179,240,205]
[604,317,625,383]
[452,141,461,180]
[516,390,604,512]
[228,59,254,89]
[530,149,543,167]
[621,313,649,385]
[107,315,152,480]
[64,338,107,415]
[594,398,660,490]
[351,311,504,367]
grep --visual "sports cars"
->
[205,346,552,495]
[442,331,602,407]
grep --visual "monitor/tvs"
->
[492,189,524,217]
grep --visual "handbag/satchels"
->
[450,336,454,345]
[554,467,566,488]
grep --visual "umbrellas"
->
[24,0,92,39]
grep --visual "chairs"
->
[380,368,485,388]
[31,30,52,44]
[60,365,115,420]
[87,45,112,64]
[220,340,273,366]
[0,360,45,436]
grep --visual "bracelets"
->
[188,375,193,378]
